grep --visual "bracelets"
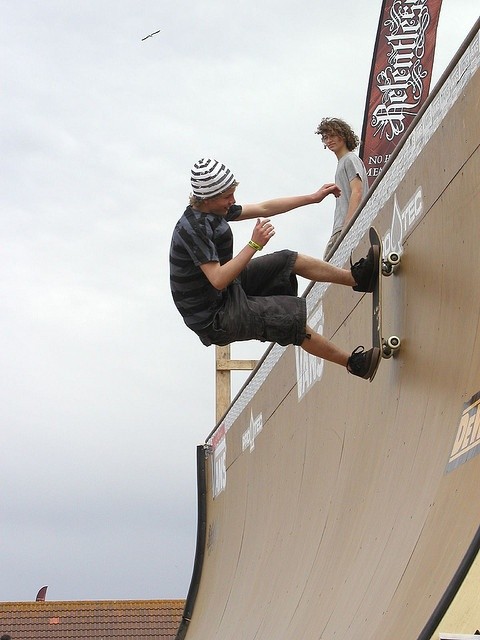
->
[248,240,263,251]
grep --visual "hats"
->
[191,158,235,199]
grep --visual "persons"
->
[314,116,369,260]
[169,157,380,380]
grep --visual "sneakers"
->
[347,345,379,379]
[350,245,379,292]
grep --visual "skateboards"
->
[368,226,401,383]
